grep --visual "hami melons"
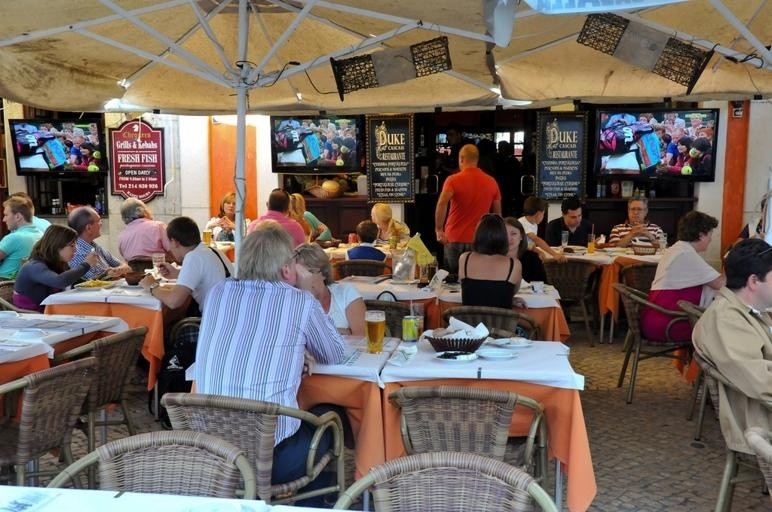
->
[322,180,340,194]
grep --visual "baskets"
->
[631,244,658,255]
[316,240,340,248]
[124,272,146,285]
[304,176,350,198]
[425,336,487,352]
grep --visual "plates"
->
[491,338,535,349]
[10,328,49,340]
[73,280,114,291]
[120,282,152,289]
[476,347,520,359]
[0,310,20,318]
[431,351,479,363]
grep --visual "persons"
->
[66,206,132,287]
[435,143,504,280]
[284,191,331,245]
[514,195,568,265]
[1,197,44,283]
[457,212,523,342]
[274,120,357,169]
[294,240,368,340]
[433,140,537,211]
[724,194,767,257]
[691,238,771,457]
[194,218,357,510]
[243,187,304,250]
[546,197,598,247]
[10,190,53,233]
[504,215,550,287]
[345,219,387,264]
[640,209,725,342]
[370,203,410,249]
[12,224,98,315]
[138,215,237,345]
[14,123,101,171]
[203,192,253,243]
[598,112,717,176]
[118,198,182,268]
[609,195,666,250]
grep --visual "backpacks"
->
[148,338,197,418]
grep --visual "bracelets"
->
[149,281,160,296]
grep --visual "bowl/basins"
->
[125,272,150,286]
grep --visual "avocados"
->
[339,180,347,186]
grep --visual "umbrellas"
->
[492,1,770,107]
[0,2,500,280]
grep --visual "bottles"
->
[419,252,429,283]
[597,175,607,199]
[392,231,422,281]
[52,199,61,215]
[427,252,439,282]
[94,195,101,215]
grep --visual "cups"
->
[561,230,569,248]
[530,281,545,292]
[587,233,596,253]
[364,310,385,355]
[152,252,165,267]
[202,228,212,247]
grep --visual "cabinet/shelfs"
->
[264,197,376,239]
[570,196,701,249]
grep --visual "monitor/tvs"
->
[9,118,109,178]
[269,114,368,176]
[592,107,719,183]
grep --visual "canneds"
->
[402,317,418,342]
[390,236,397,249]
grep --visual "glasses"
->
[289,249,301,261]
[628,207,643,212]
[757,246,771,256]
